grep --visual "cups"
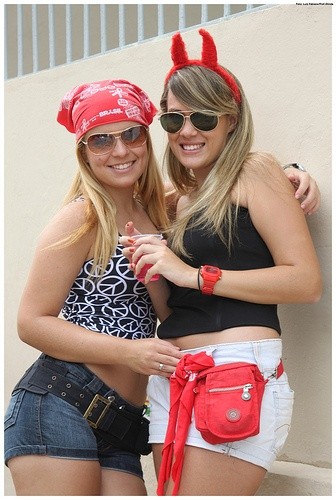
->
[130,233,163,281]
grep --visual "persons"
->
[118,28,320,496]
[4,79,320,496]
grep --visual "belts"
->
[28,365,142,442]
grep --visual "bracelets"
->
[197,267,202,291]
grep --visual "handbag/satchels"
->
[193,361,264,445]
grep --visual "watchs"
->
[281,163,306,172]
[199,265,222,296]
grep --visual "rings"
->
[159,364,163,371]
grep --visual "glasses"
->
[81,125,150,156]
[157,109,220,134]
